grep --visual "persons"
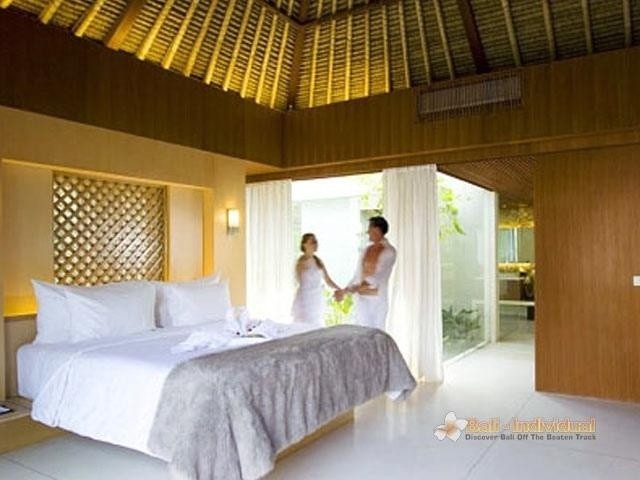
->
[293,232,340,328]
[332,217,397,330]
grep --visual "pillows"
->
[32,272,231,342]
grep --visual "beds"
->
[2,315,385,480]
